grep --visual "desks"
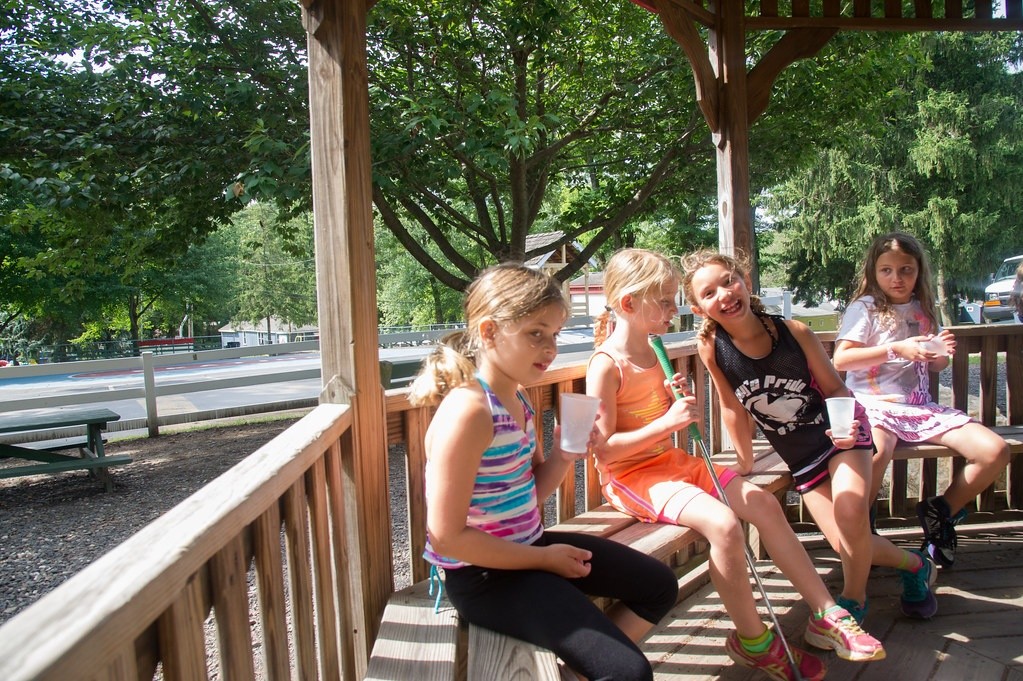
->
[0,407,121,484]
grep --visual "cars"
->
[981,253,1022,324]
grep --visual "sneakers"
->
[837,592,868,631]
[899,550,940,620]
[806,607,887,662]
[727,628,825,681]
[915,494,956,565]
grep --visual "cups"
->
[560,393,602,454]
[918,335,951,356]
[825,397,855,439]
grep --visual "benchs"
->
[1,434,108,470]
[0,452,133,496]
[1,322,1022,680]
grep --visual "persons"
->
[406,261,679,681]
[676,242,938,629]
[1009,261,1023,323]
[831,231,1012,572]
[582,245,889,681]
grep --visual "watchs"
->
[886,342,898,361]
